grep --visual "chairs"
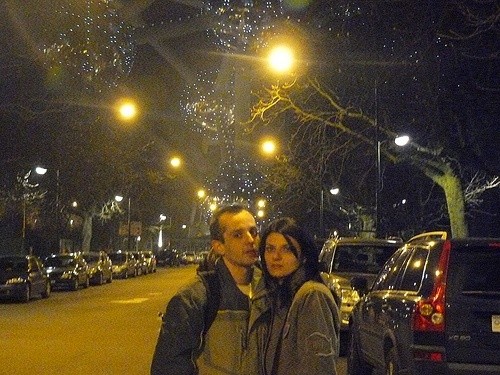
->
[2,262,14,270]
[339,260,364,273]
[17,262,26,269]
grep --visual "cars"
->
[43,251,90,292]
[109,249,211,280]
[83,251,113,286]
[0,255,52,304]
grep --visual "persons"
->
[150,204,262,375]
[251,217,342,375]
[422,231,433,240]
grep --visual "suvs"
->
[346,229,500,375]
[318,230,410,334]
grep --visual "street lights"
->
[373,133,415,236]
